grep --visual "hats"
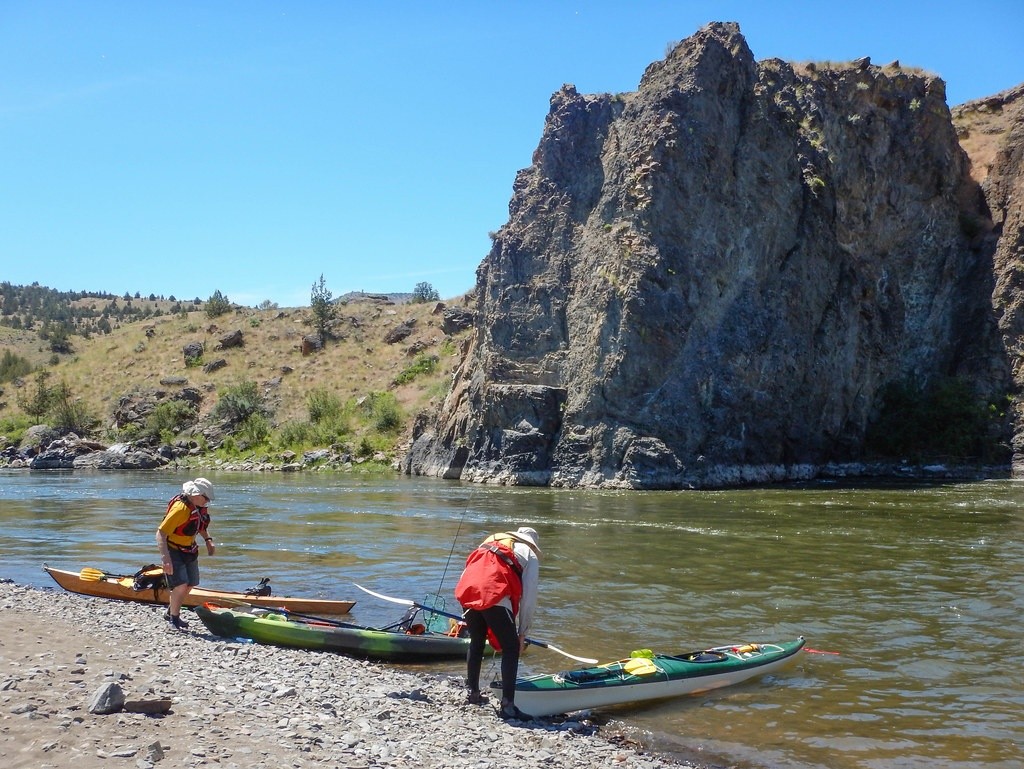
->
[183,477,215,500]
[505,526,542,553]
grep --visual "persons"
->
[155,477,215,628]
[454,527,541,721]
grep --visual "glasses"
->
[201,495,210,502]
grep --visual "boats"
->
[41,563,357,615]
[489,636,807,717]
[193,600,529,658]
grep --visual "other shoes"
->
[498,701,534,722]
[467,694,490,705]
[168,613,189,628]
[163,605,171,621]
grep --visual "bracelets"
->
[205,537,212,541]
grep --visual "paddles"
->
[77,569,141,583]
[218,596,404,633]
[349,579,599,666]
